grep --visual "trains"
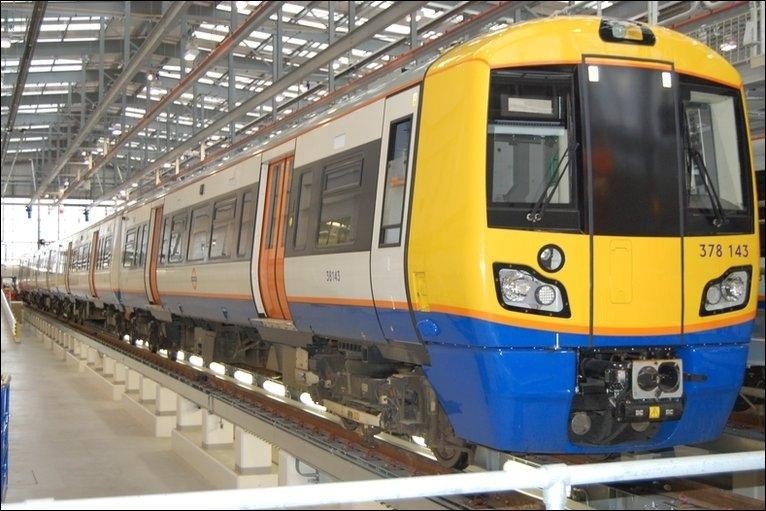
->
[16,14,762,473]
